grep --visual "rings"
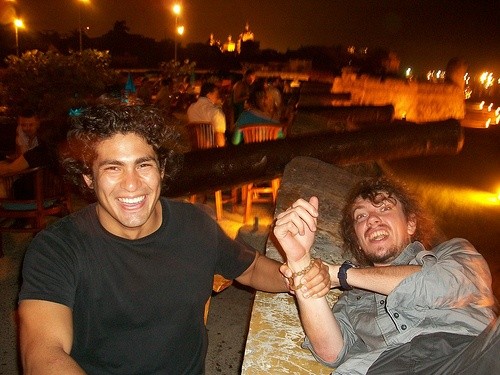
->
[291,204,294,209]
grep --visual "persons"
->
[273,175,500,375]
[0,47,298,228]
[19,102,331,375]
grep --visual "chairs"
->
[0,166,73,237]
[185,121,283,224]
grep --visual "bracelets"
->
[338,260,361,290]
[288,260,315,290]
